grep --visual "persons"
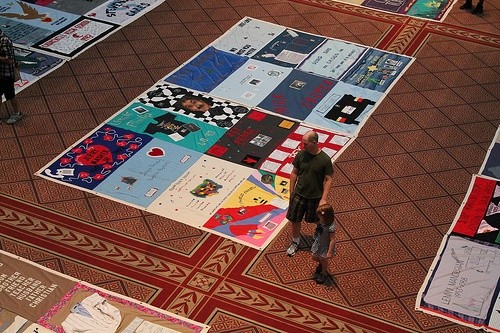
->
[311,204,336,284]
[459,0,484,15]
[0,29,23,125]
[286,130,334,257]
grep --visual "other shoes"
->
[316,264,322,274]
[459,2,472,10]
[471,6,483,14]
[317,274,327,284]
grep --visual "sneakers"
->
[6,111,22,123]
[287,242,301,256]
[311,237,320,253]
